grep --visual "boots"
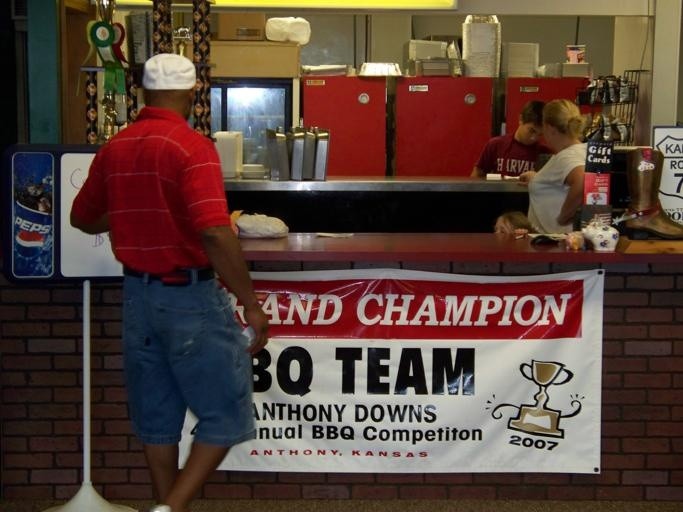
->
[623,148,683,240]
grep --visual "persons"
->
[493,210,540,235]
[516,99,588,235]
[470,100,558,177]
[70,53,271,512]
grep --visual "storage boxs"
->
[183,11,301,80]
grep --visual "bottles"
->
[14,198,53,262]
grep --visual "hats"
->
[142,52,196,91]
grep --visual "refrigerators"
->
[193,76,302,143]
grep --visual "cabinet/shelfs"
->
[574,83,638,147]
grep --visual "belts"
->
[122,264,215,287]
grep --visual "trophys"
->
[492,357,582,440]
